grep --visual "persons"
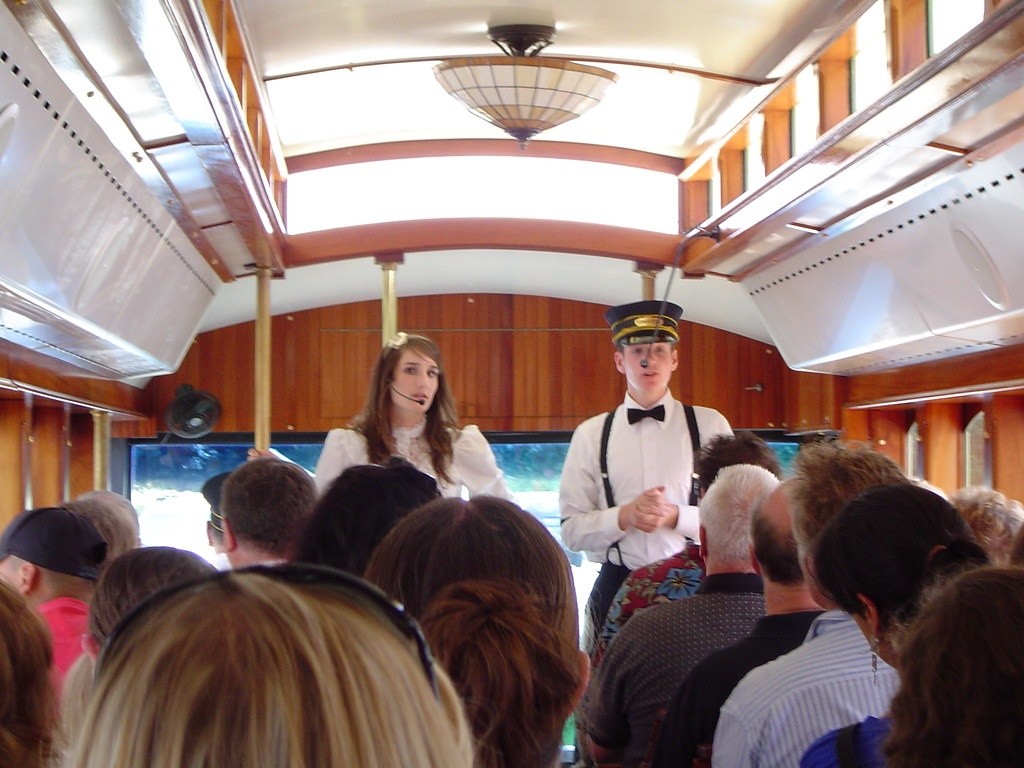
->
[574,433,1024,768]
[559,300,735,648]
[0,457,590,768]
[313,332,509,499]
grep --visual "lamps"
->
[432,14,620,151]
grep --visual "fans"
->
[164,383,220,439]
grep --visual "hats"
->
[0,507,108,584]
[200,472,235,534]
[601,300,683,347]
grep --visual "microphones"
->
[638,227,719,368]
[387,382,425,407]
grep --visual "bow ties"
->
[627,404,666,426]
